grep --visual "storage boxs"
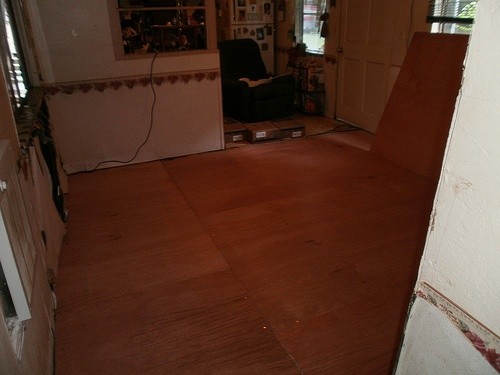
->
[224,124,247,143]
[242,122,280,144]
[285,63,324,93]
[298,92,325,115]
[273,121,305,139]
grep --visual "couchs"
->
[219,37,296,124]
